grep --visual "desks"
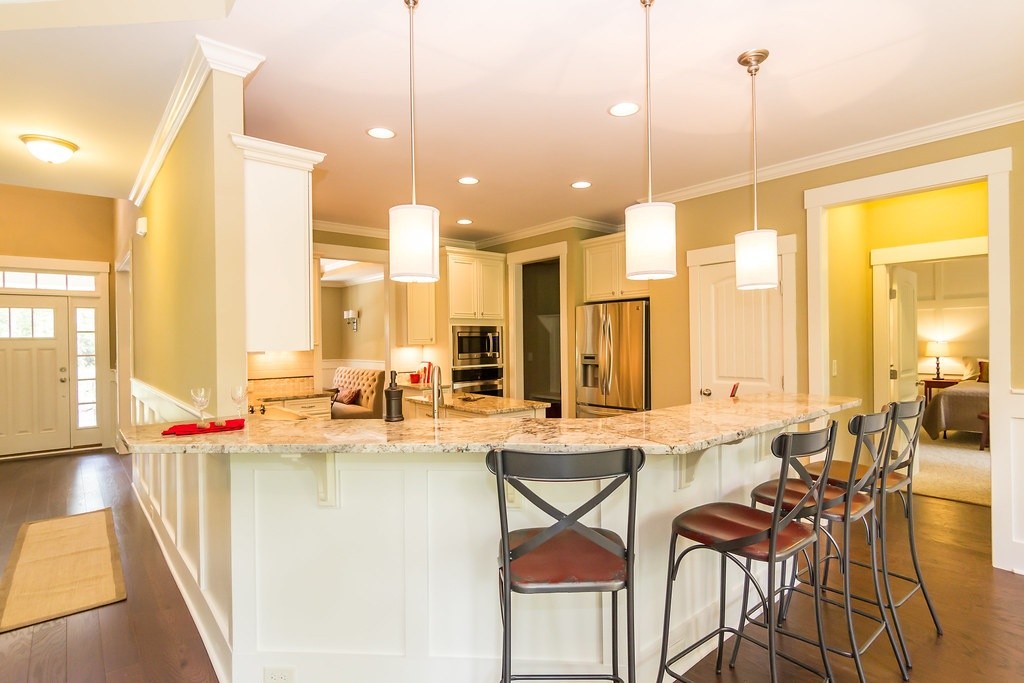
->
[923,378,961,407]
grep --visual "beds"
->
[327,367,385,419]
[921,380,989,440]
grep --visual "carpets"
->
[898,425,993,507]
[0,507,127,633]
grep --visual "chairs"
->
[485,445,646,683]
[803,394,943,668]
[655,418,839,682]
[729,407,912,682]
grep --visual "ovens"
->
[451,367,504,397]
[452,325,503,366]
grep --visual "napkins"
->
[160,417,245,437]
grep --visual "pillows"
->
[332,388,360,403]
[961,355,989,382]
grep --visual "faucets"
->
[432,365,445,420]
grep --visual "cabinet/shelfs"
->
[398,247,507,346]
[582,231,649,302]
[231,134,327,351]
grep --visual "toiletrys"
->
[385,369,404,421]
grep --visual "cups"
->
[410,373,422,383]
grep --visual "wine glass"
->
[190,386,211,428]
[229,383,247,419]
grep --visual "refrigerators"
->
[575,300,650,418]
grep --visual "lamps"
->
[735,50,778,291]
[624,0,678,280]
[344,310,359,332]
[18,134,80,164]
[387,1,440,283]
[925,341,949,380]
